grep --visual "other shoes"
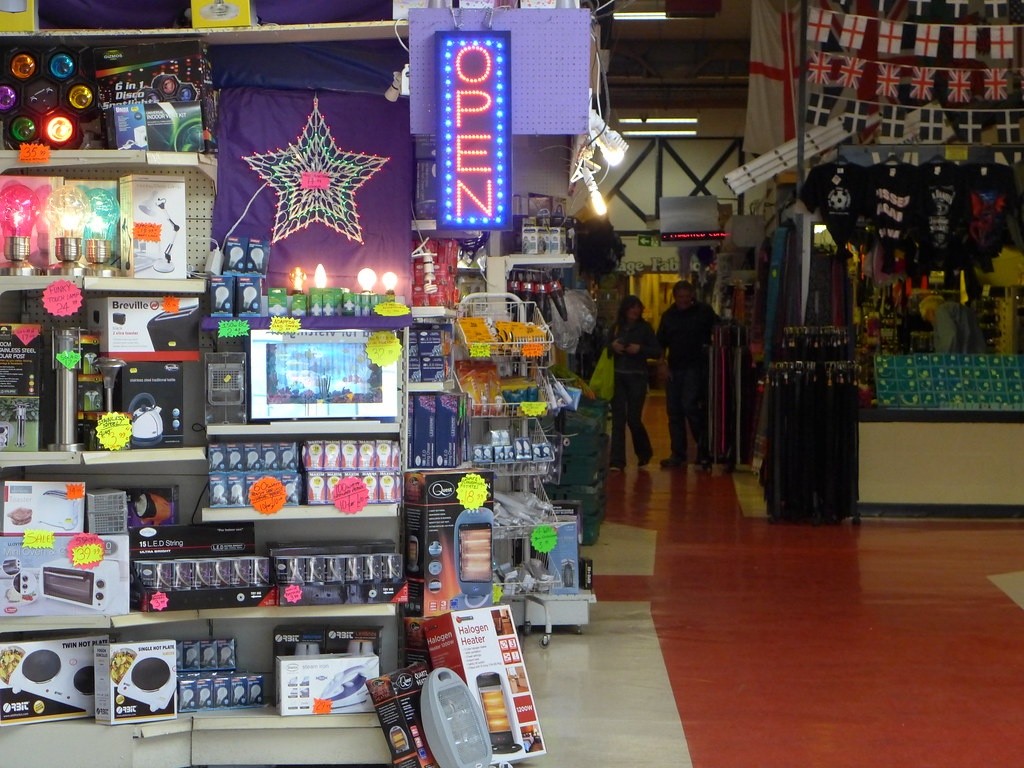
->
[660,452,688,469]
[637,450,654,468]
[609,458,627,472]
[693,450,713,471]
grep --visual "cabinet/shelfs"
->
[0,149,459,666]
[455,296,597,634]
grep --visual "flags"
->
[800,0,1024,150]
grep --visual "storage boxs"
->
[0,323,46,452]
[524,227,567,255]
[397,323,454,383]
[1,0,42,31]
[412,134,437,220]
[0,174,188,279]
[0,624,386,768]
[527,192,567,227]
[413,237,459,308]
[367,661,441,768]
[579,557,593,589]
[202,351,247,426]
[93,39,220,153]
[267,287,386,317]
[402,467,494,619]
[408,391,469,468]
[543,397,609,546]
[472,429,552,461]
[75,331,132,451]
[104,361,184,449]
[456,360,537,417]
[87,296,201,362]
[191,0,259,29]
[546,500,584,544]
[404,616,432,674]
[0,480,408,616]
[421,605,547,766]
[210,237,271,317]
[545,522,578,593]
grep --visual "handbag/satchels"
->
[589,346,615,402]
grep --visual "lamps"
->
[384,71,401,102]
[569,89,629,217]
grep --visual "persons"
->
[919,295,988,354]
[607,294,656,471]
[656,280,723,472]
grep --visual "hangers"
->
[883,145,902,167]
[832,145,849,168]
[929,145,947,164]
